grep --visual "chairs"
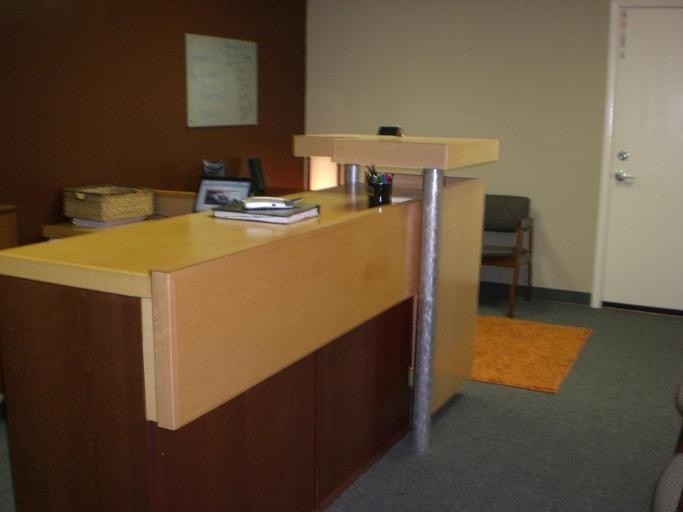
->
[482,192,534,316]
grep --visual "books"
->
[211,200,321,225]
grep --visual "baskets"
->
[60,184,155,221]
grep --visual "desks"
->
[140,181,309,218]
[41,216,150,239]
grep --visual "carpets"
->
[471,315,592,394]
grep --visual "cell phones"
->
[242,196,303,210]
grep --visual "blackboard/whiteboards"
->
[185,33,258,127]
